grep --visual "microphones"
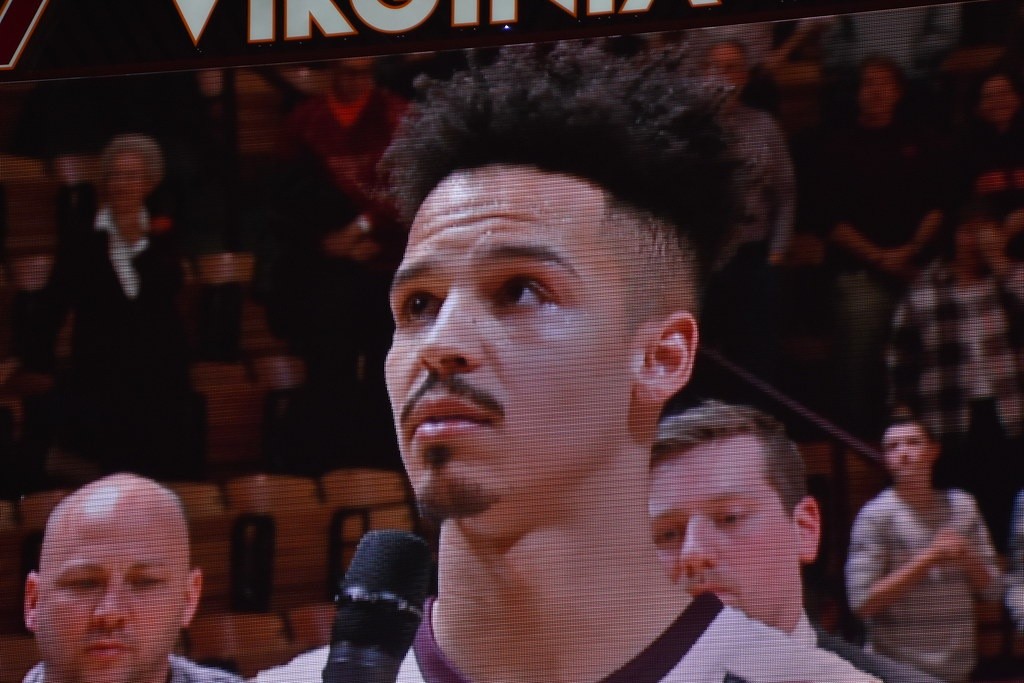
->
[321,528,434,683]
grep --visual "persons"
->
[234,46,943,683]
[847,408,1004,683]
[2,50,419,474]
[16,474,242,683]
[693,38,1024,409]
[648,401,948,683]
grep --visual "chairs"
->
[0,68,419,683]
